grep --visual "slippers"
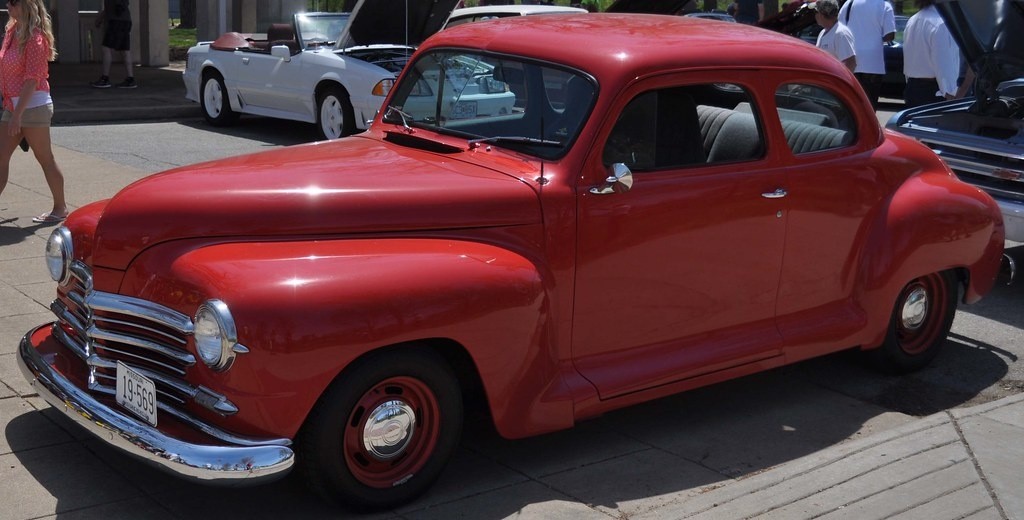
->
[33,213,66,223]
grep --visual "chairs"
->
[541,73,709,170]
[254,21,366,56]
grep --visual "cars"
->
[683,12,740,24]
[784,13,978,101]
[881,0,1024,285]
[15,10,1009,514]
[444,3,588,91]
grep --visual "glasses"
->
[10,0,18,5]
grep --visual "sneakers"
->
[116,78,138,89]
[88,77,112,88]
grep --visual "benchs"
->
[696,104,857,168]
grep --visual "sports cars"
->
[180,0,526,142]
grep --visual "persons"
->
[808,0,858,73]
[0,1,69,223]
[837,0,897,113]
[779,0,805,16]
[89,0,137,89]
[901,0,975,110]
[727,0,765,27]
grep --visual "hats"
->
[808,0,839,15]
